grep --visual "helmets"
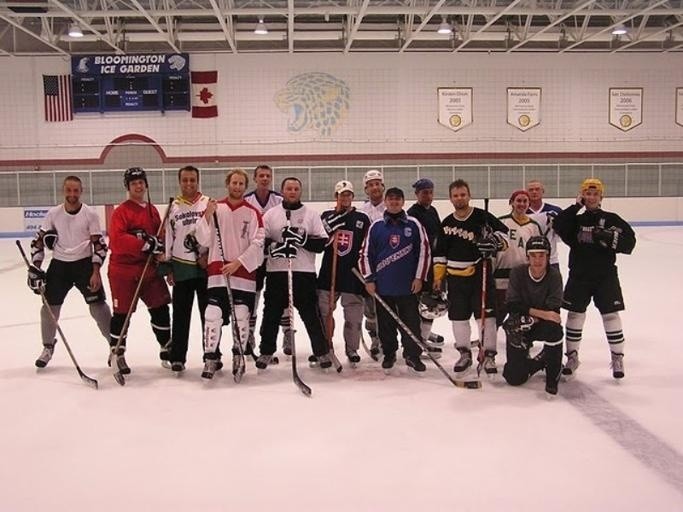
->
[362,170,383,186]
[123,167,148,190]
[580,179,603,199]
[418,290,450,319]
[525,235,550,257]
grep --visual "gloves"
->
[269,226,308,258]
[131,229,163,254]
[591,227,618,250]
[27,263,46,295]
[502,315,533,351]
[476,234,501,258]
[43,230,57,250]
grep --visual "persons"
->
[553,178,636,377]
[255,177,331,369]
[165,165,222,371]
[194,169,265,379]
[243,164,297,355]
[308,180,565,395]
[108,167,171,375]
[27,176,112,367]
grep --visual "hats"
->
[412,178,432,192]
[334,180,354,198]
[385,188,403,201]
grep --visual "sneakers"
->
[482,350,497,373]
[159,347,184,371]
[108,354,131,375]
[610,351,624,378]
[307,349,359,368]
[533,350,577,394]
[202,330,272,379]
[35,339,57,367]
[283,329,296,355]
[406,333,443,372]
[453,343,471,372]
[368,330,395,368]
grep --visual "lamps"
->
[68,21,83,37]
[254,16,267,34]
[437,16,452,33]
[611,24,628,35]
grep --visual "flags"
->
[43,74,73,122]
[192,69,218,118]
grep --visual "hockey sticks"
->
[212,211,245,383]
[352,267,483,389]
[285,210,311,398]
[111,197,173,386]
[327,199,343,373]
[476,197,489,377]
[16,239,97,389]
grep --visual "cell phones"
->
[576,192,586,205]
